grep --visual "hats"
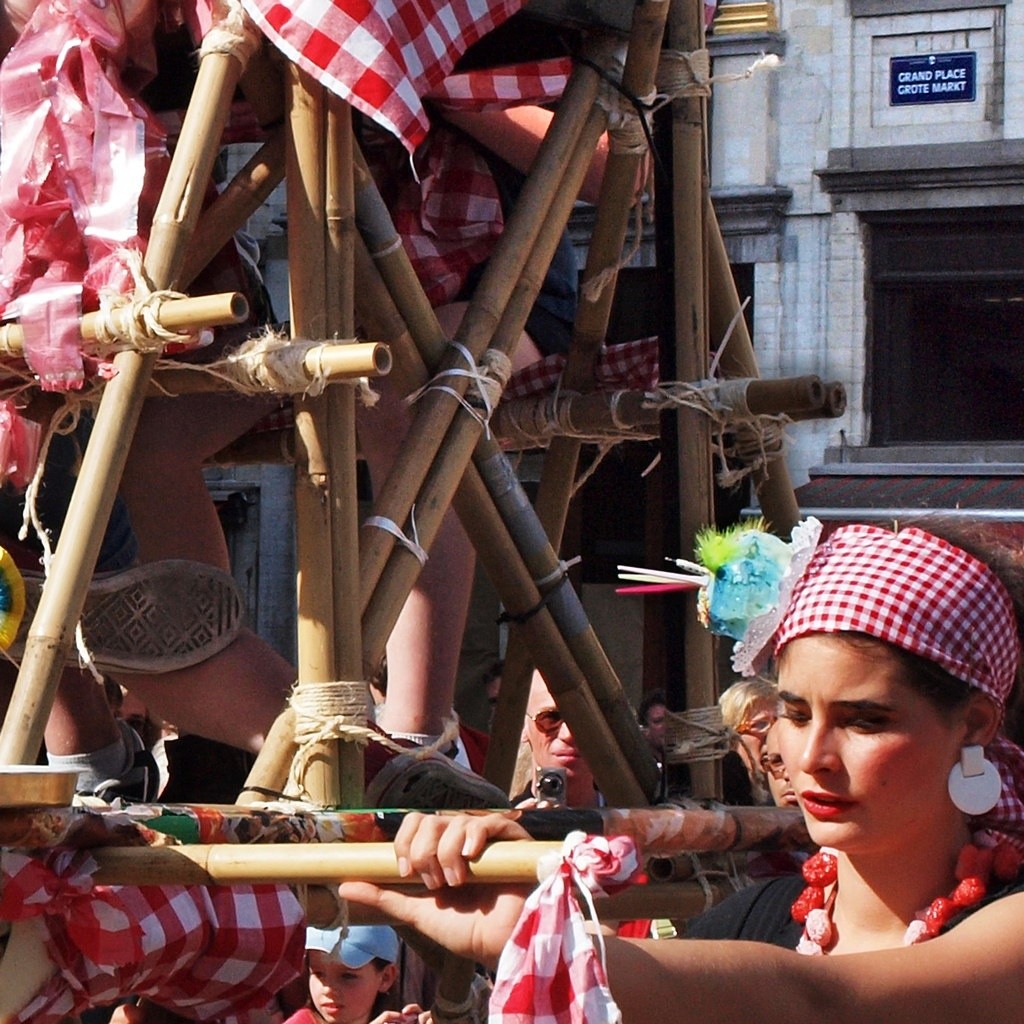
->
[305,925,398,967]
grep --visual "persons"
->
[0,0,1024,1024]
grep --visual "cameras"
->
[535,765,566,809]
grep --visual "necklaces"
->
[792,840,1021,957]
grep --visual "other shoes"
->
[4,560,246,672]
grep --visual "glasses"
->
[526,708,564,735]
[760,755,787,772]
[736,718,775,735]
